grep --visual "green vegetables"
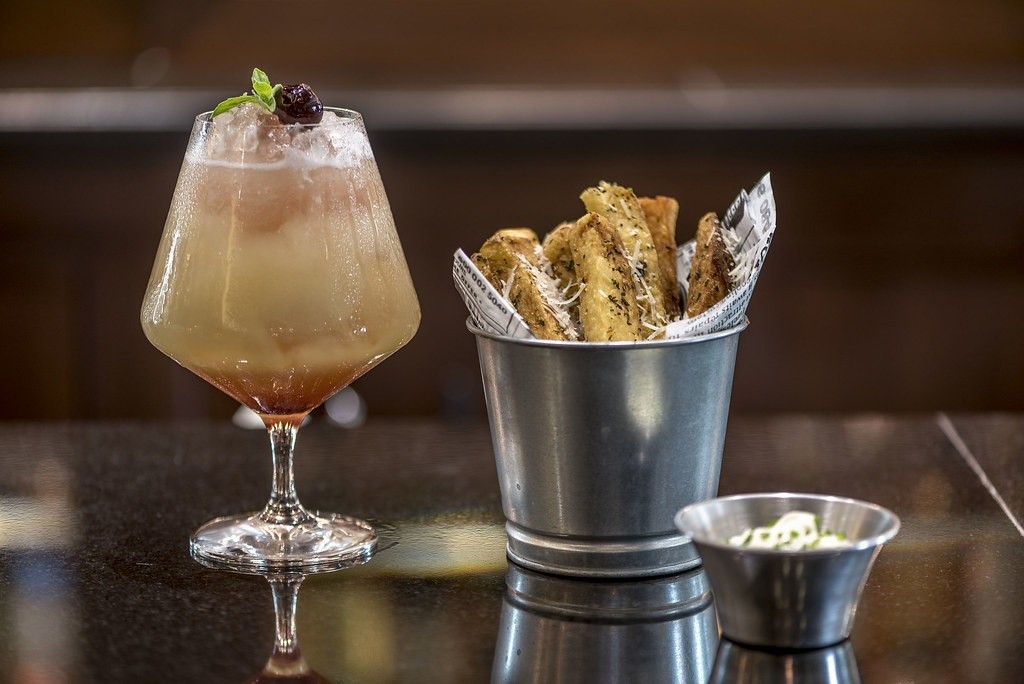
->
[208,66,283,120]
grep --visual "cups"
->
[466,314,749,577]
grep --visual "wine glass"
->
[141,106,421,566]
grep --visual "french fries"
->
[472,183,731,344]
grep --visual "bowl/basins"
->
[674,493,901,647]
